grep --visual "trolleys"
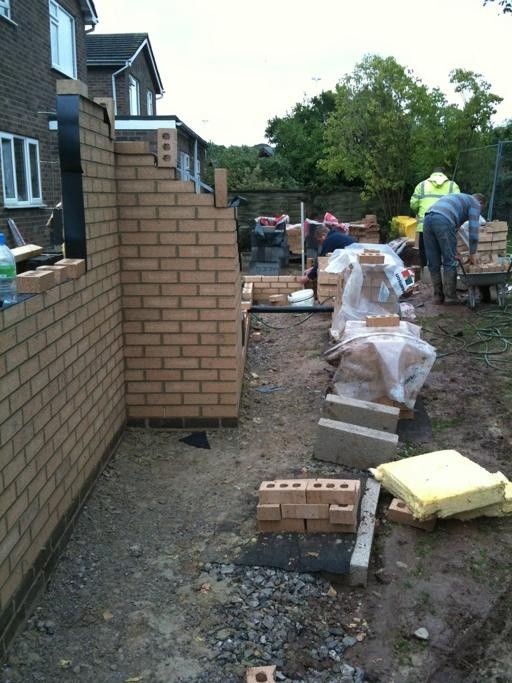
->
[453,254,512,309]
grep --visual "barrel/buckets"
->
[287,289,313,307]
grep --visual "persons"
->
[410,167,461,264]
[298,226,358,285]
[422,191,487,304]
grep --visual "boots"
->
[444,270,467,305]
[431,273,444,304]
